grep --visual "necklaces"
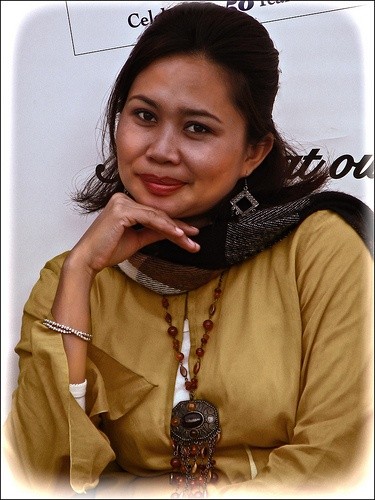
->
[158,272,226,499]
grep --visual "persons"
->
[2,2,375,498]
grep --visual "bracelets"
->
[42,318,93,344]
